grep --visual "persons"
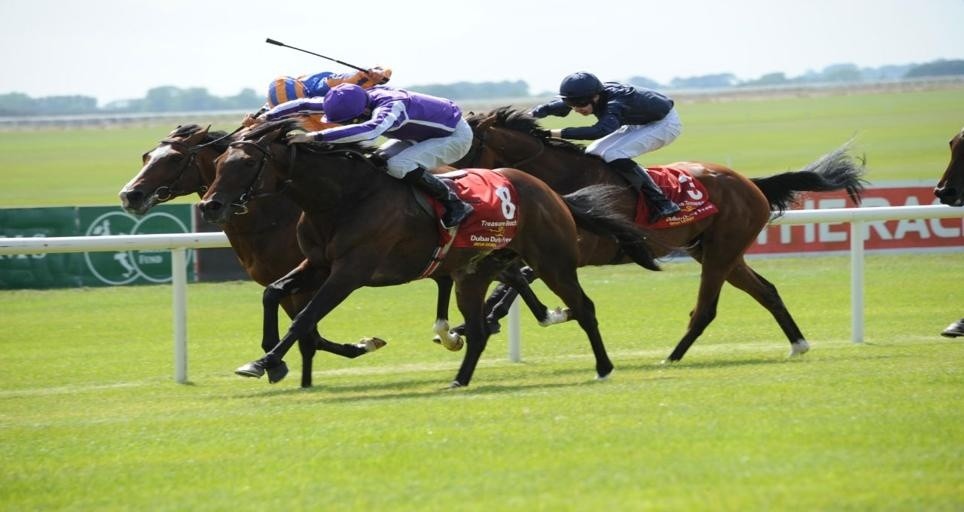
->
[287,80,474,225]
[240,67,392,141]
[530,69,682,224]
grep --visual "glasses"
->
[563,99,590,107]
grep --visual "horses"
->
[444,104,873,395]
[930,132,964,338]
[115,104,664,392]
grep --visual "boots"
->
[400,164,474,227]
[606,158,681,224]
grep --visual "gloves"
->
[282,129,322,146]
[526,128,551,138]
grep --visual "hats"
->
[553,71,598,98]
[268,78,310,110]
[320,83,367,124]
